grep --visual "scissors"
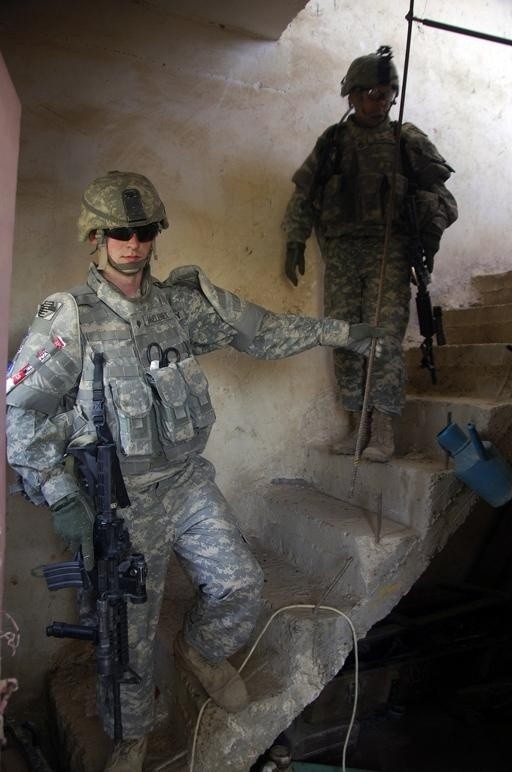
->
[146,342,181,369]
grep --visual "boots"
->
[362,410,396,463]
[171,630,249,713]
[332,410,370,457]
[103,735,147,772]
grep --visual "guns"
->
[44,441,146,745]
[404,191,446,385]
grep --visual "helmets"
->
[77,171,169,242]
[340,52,400,97]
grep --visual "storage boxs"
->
[308,659,400,724]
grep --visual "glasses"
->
[356,86,398,104]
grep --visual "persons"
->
[283,46,457,461]
[6,167,385,772]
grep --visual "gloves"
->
[419,222,444,273]
[49,491,97,571]
[283,240,308,286]
[345,322,387,361]
